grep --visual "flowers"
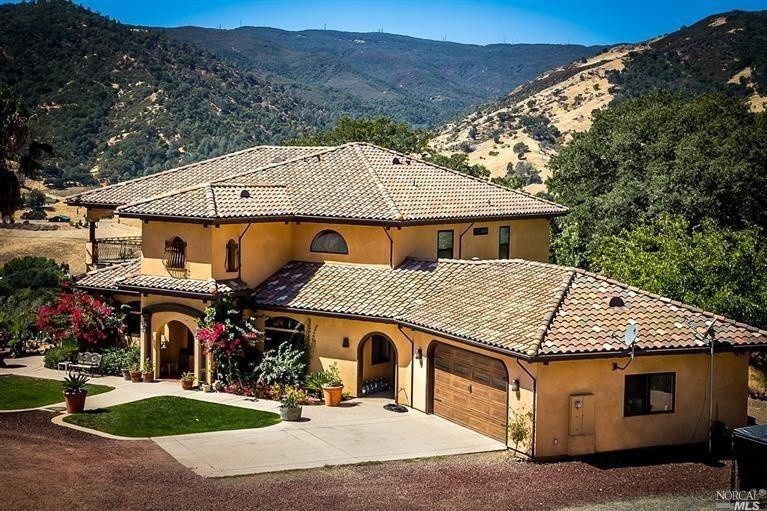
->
[280,383,306,407]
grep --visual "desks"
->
[163,360,177,376]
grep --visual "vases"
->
[279,404,302,420]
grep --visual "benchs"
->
[58,350,103,378]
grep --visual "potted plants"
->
[59,367,92,413]
[120,356,155,382]
[320,361,344,405]
[180,367,212,392]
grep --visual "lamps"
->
[512,376,520,390]
[415,348,422,358]
